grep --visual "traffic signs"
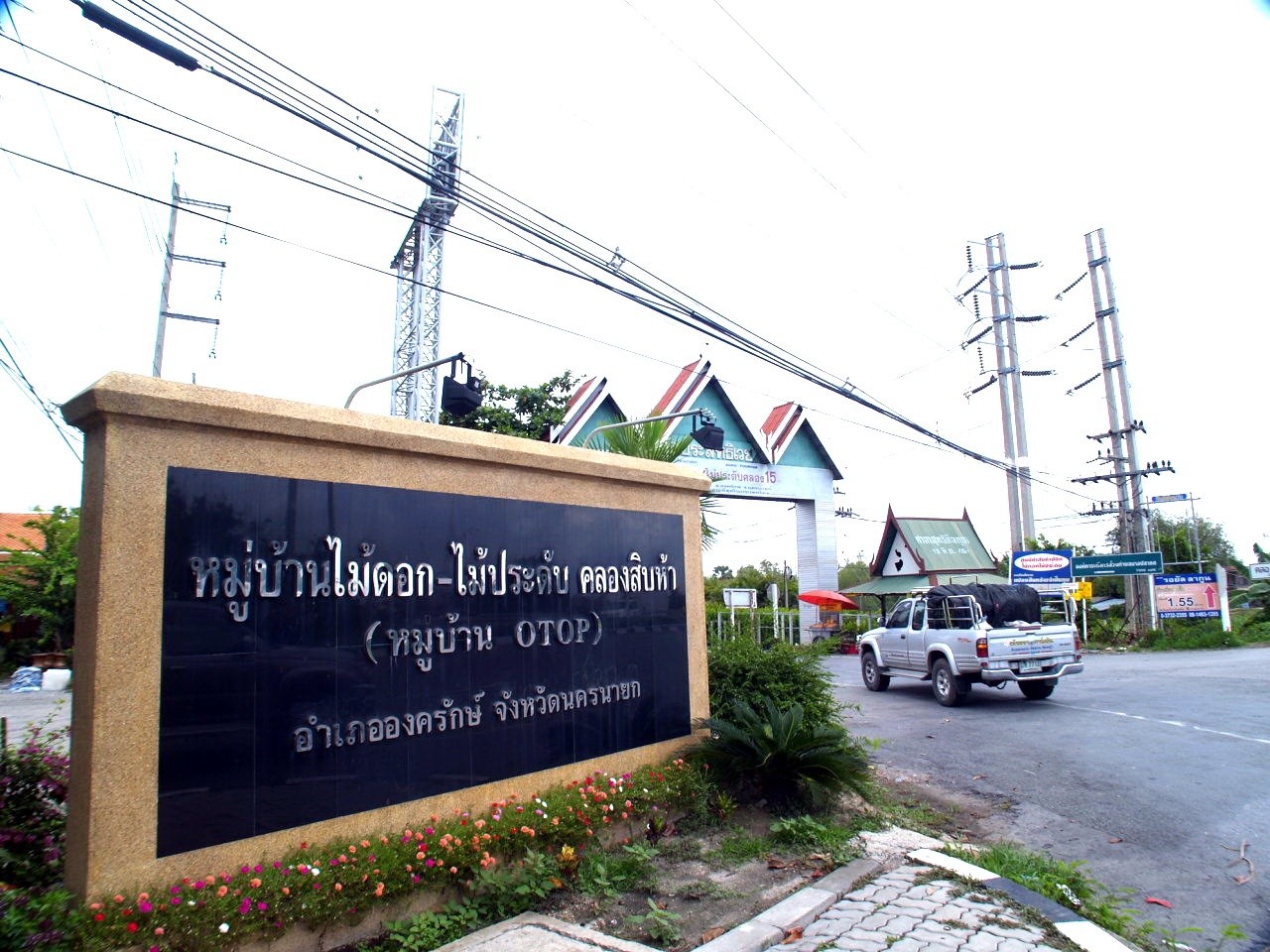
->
[1152,573,1221,619]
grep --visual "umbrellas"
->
[797,589,860,623]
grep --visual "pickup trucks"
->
[855,586,1084,709]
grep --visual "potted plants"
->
[833,621,867,654]
